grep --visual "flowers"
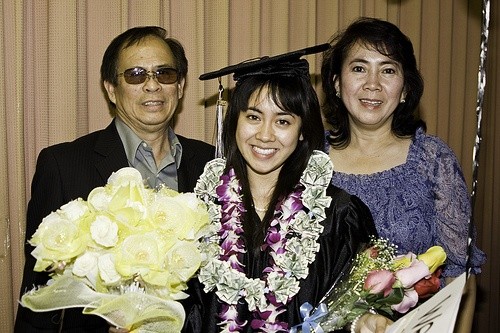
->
[17,167,220,333]
[215,169,304,333]
[193,149,334,311]
[289,236,472,333]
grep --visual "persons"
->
[318,17,488,333]
[188,44,395,333]
[13,26,220,333]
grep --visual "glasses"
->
[107,67,181,85]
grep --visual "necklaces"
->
[193,149,333,333]
[255,205,268,213]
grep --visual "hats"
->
[199,42,331,160]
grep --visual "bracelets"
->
[350,312,366,333]
